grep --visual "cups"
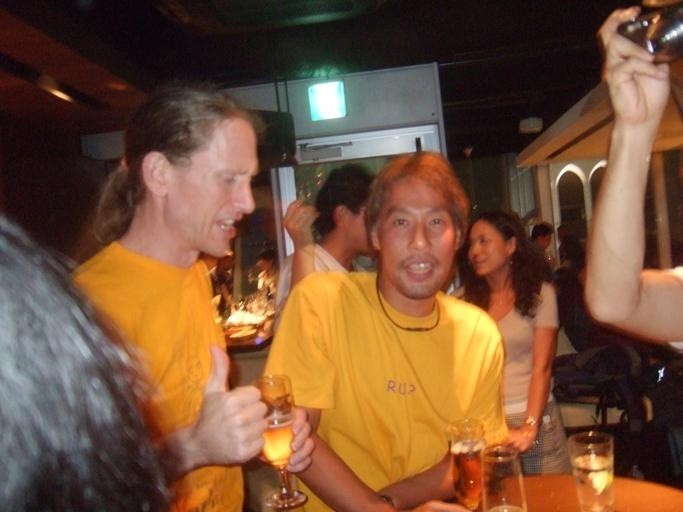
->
[567,430,617,511]
[617,0,683,63]
[481,445,527,512]
[220,286,277,341]
[447,418,489,511]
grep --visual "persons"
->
[440,211,572,476]
[68,86,316,510]
[258,152,508,511]
[527,221,552,255]
[556,220,631,350]
[0,214,173,512]
[209,250,235,314]
[584,2,682,352]
[254,247,277,298]
[270,164,375,336]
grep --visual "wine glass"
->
[250,374,308,510]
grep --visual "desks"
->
[448,472,683,512]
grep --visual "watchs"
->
[524,413,539,429]
